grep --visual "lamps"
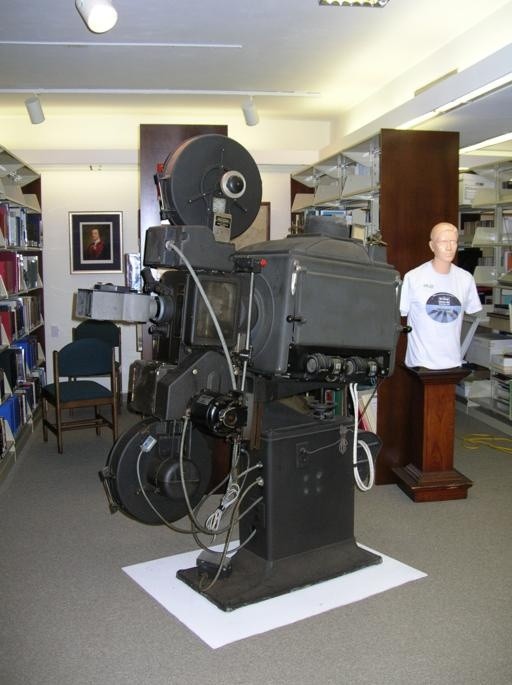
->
[26,95,46,127]
[75,0,118,35]
[239,96,261,128]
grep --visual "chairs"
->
[39,340,118,455]
[68,319,123,416]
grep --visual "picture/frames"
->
[68,211,124,275]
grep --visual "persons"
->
[85,228,110,261]
[400,223,482,369]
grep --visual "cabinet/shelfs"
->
[0,144,46,485]
[290,127,473,504]
[460,161,512,436]
